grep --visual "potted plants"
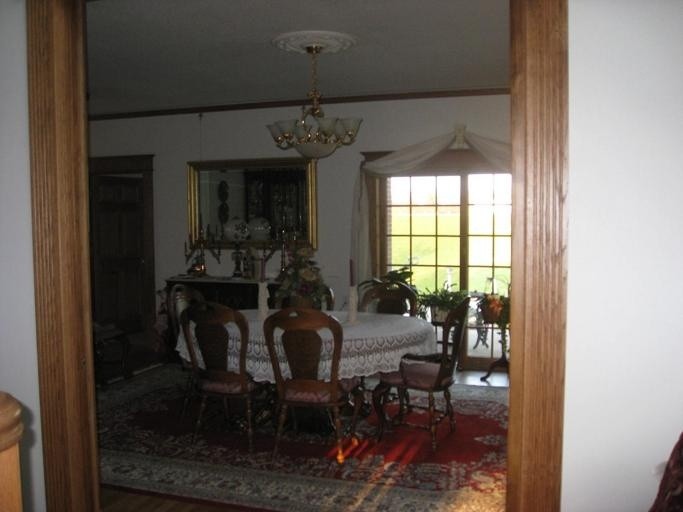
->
[473,290,514,354]
[357,268,418,315]
[416,280,471,324]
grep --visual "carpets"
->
[84,365,511,512]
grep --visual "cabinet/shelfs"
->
[163,270,317,361]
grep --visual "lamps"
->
[266,30,364,162]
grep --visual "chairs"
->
[360,282,418,417]
[265,306,364,467]
[179,300,279,457]
[373,298,469,450]
[169,282,230,426]
[281,282,336,310]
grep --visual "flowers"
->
[278,246,330,311]
